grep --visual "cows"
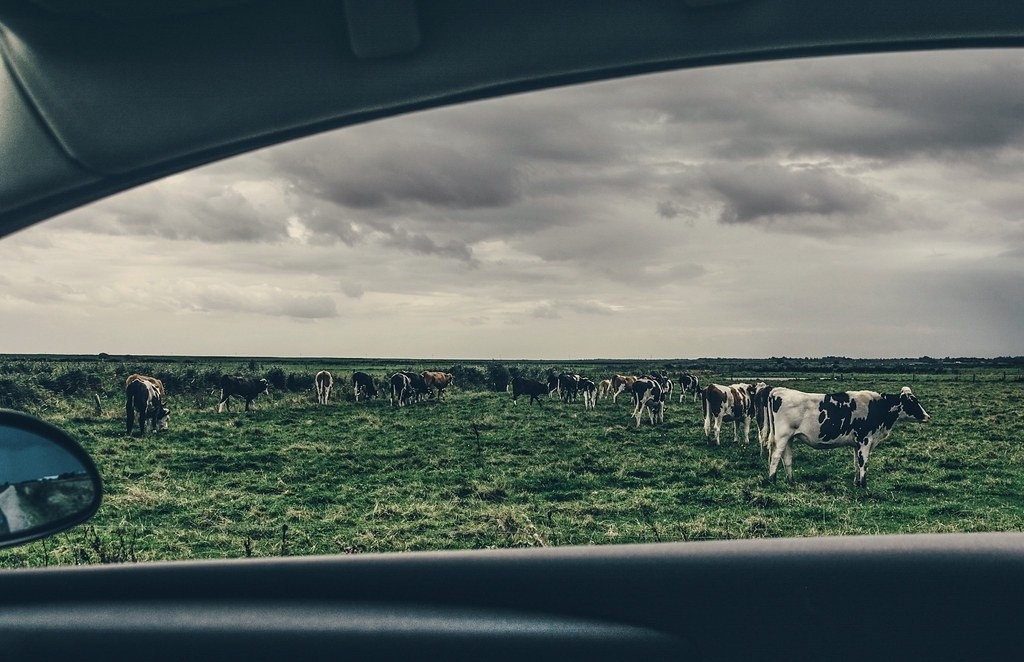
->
[513,369,703,429]
[125,373,170,437]
[352,372,379,401]
[314,370,334,406]
[701,382,931,489]
[218,378,269,413]
[390,371,455,407]
